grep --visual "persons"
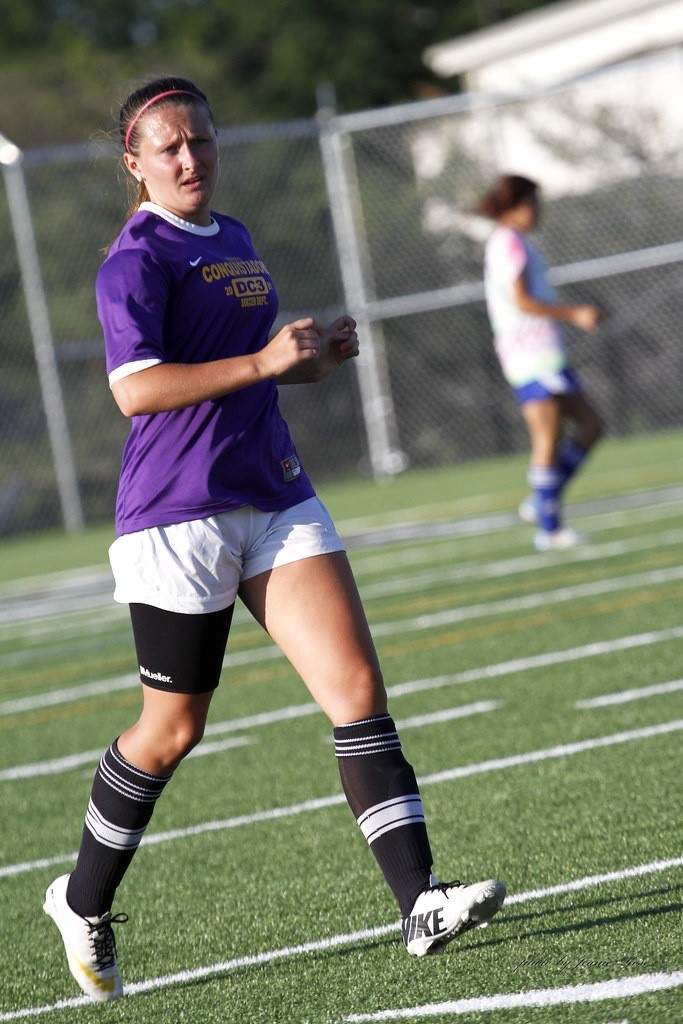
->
[481,175,607,549]
[37,74,506,1000]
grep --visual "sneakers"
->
[399,873,505,957]
[42,873,131,1001]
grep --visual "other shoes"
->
[535,528,584,550]
[518,497,539,524]
